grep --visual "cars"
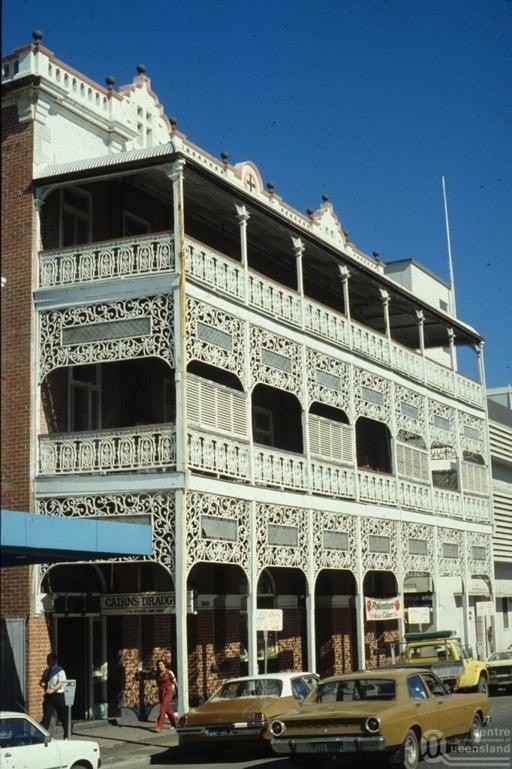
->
[176,630,512,768]
[0,711,102,769]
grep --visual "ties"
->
[44,668,52,691]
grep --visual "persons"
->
[148,660,179,733]
[39,653,72,740]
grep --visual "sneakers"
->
[149,726,176,733]
